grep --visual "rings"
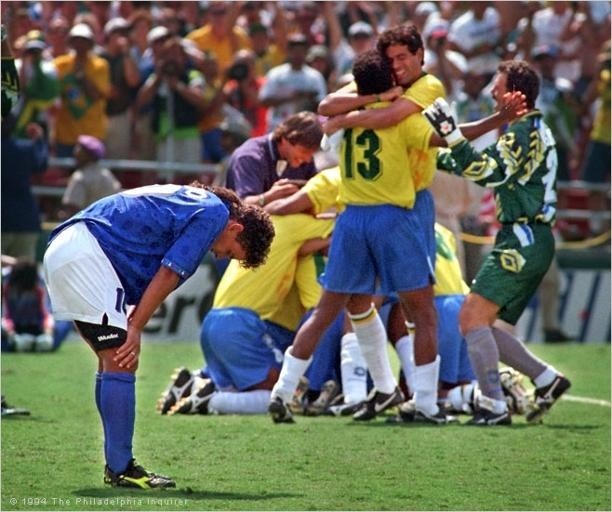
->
[130,352,136,356]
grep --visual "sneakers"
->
[2,396,31,416]
[156,366,192,414]
[269,372,570,427]
[106,458,176,490]
[165,378,215,414]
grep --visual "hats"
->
[25,3,562,67]
[78,134,105,161]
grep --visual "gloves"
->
[14,334,32,351]
[34,334,52,351]
[421,98,465,145]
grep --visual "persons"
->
[41,182,276,491]
[1,2,612,427]
